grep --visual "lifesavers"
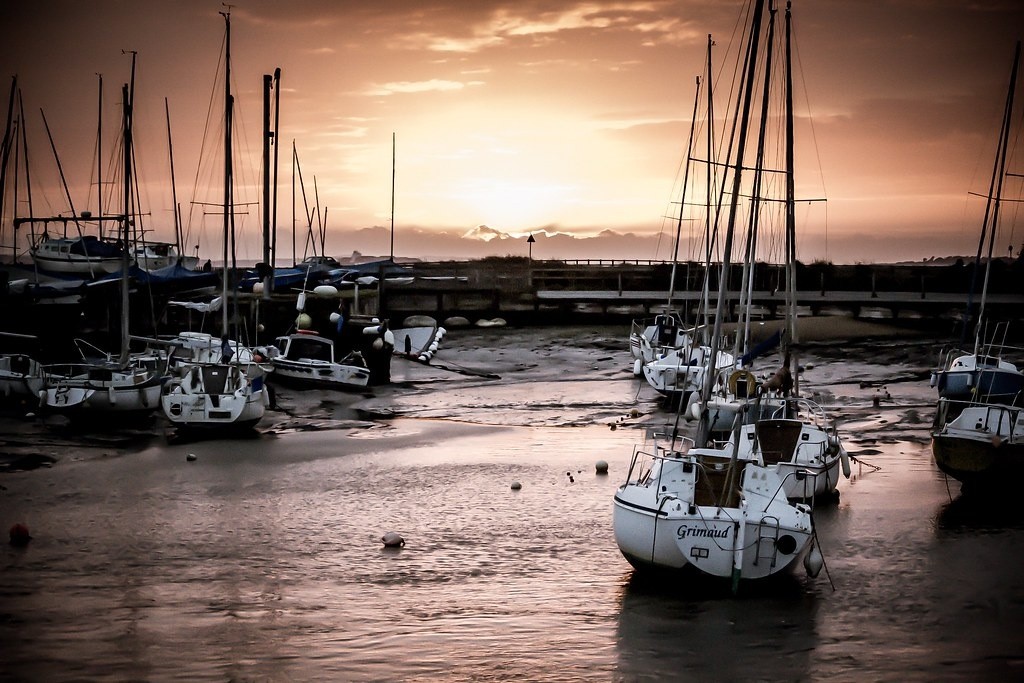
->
[728,369,757,398]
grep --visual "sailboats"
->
[930,28,1024,488]
[0,1,447,444]
[612,1,852,601]
